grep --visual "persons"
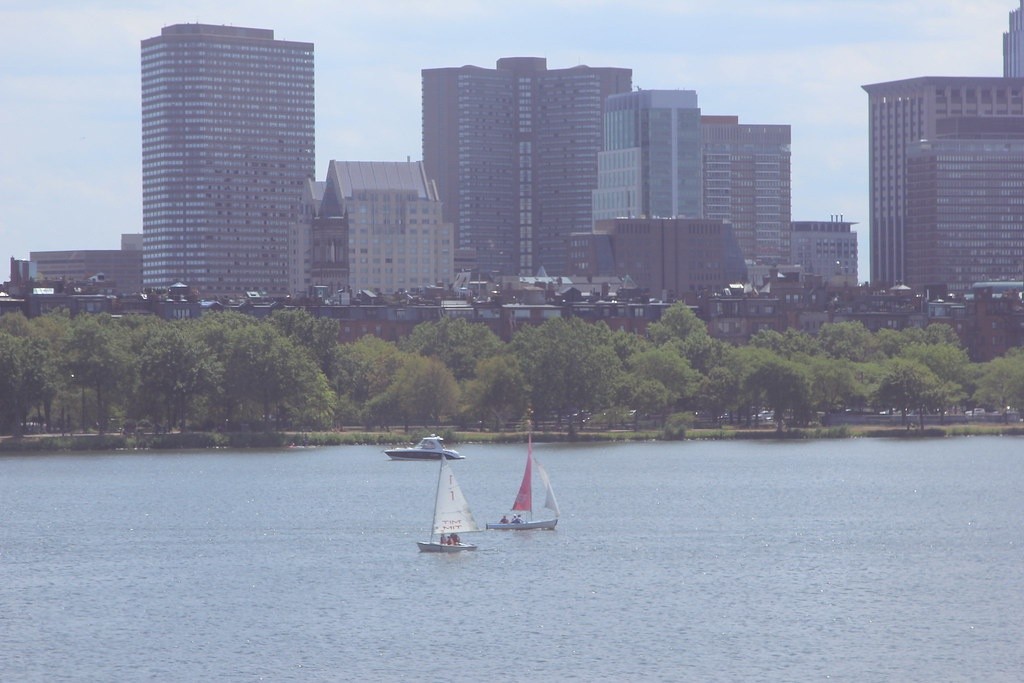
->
[441,533,461,545]
[515,515,524,524]
[512,514,516,521]
[498,515,509,524]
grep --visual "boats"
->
[381,433,467,460]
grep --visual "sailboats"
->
[415,452,483,554]
[483,418,561,531]
